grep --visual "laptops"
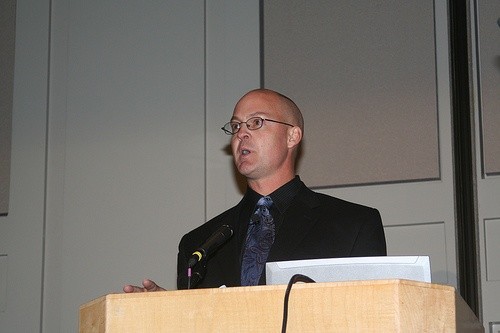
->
[266,256,432,287]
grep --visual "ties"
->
[241,196,275,286]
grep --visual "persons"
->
[122,89,390,294]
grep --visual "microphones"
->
[189,224,235,268]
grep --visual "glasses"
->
[221,117,294,134]
